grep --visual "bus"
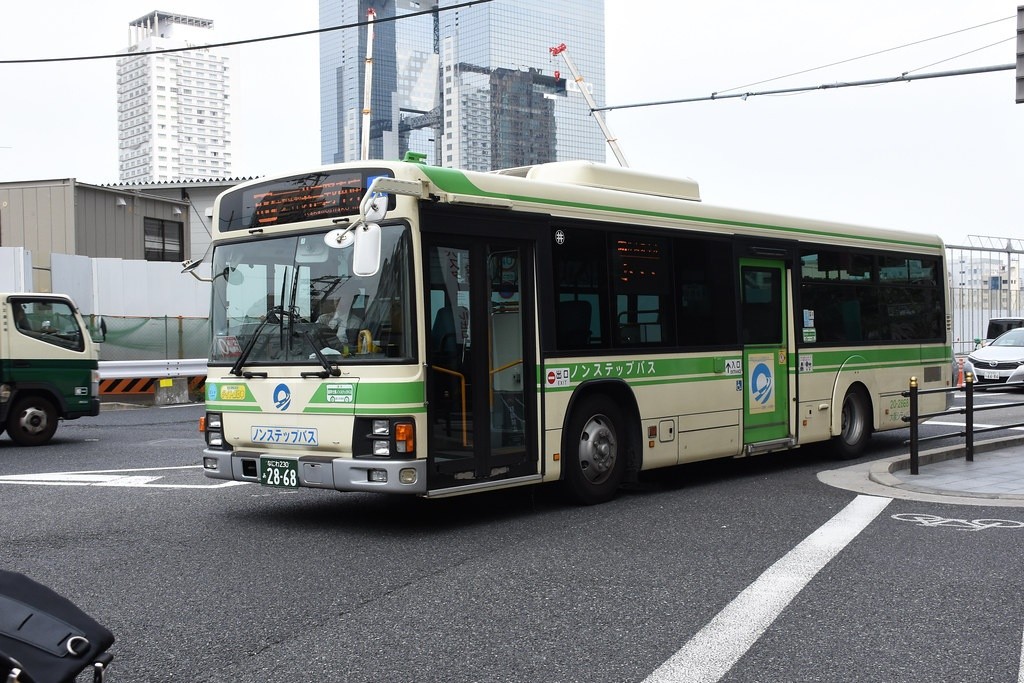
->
[178,157,961,510]
[985,318,1024,348]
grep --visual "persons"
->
[15,307,46,333]
[315,286,363,343]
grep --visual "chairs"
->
[554,300,590,348]
[430,303,470,361]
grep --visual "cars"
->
[962,327,1024,395]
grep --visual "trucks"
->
[0,291,108,448]
